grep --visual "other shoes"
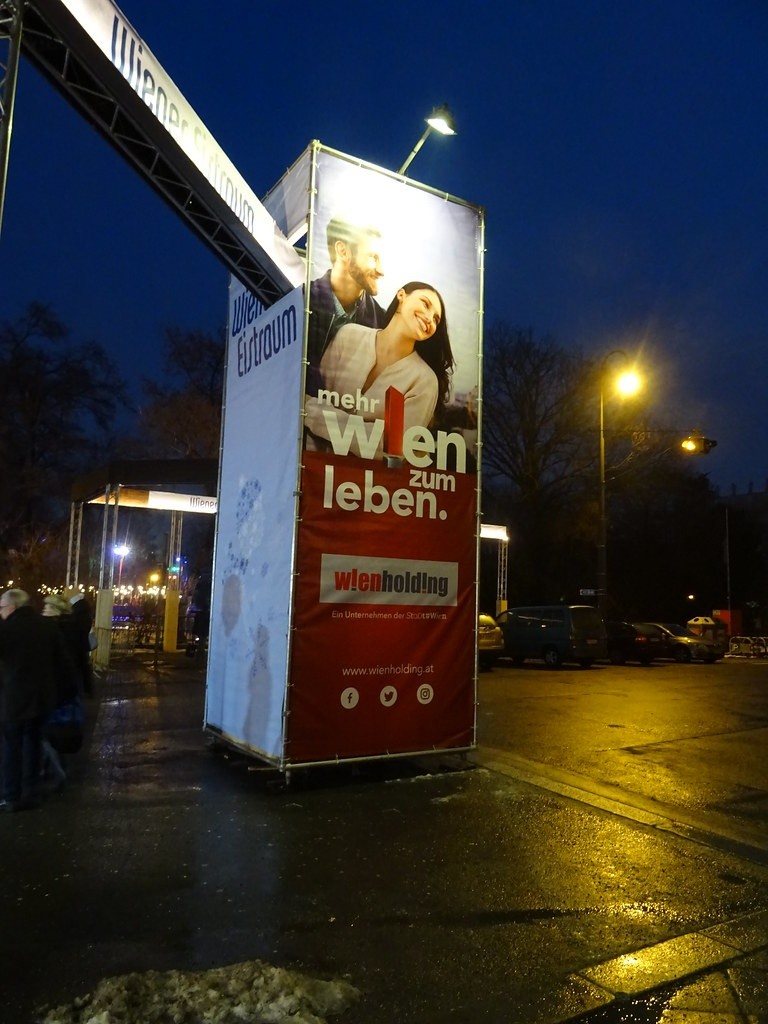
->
[0,799,18,811]
[21,784,34,796]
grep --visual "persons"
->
[304,210,389,450]
[305,282,457,464]
[0,589,96,812]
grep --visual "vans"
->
[492,605,608,671]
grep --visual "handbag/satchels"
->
[44,719,82,753]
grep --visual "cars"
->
[631,622,720,663]
[477,612,504,671]
[605,618,672,666]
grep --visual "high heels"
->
[47,769,71,793]
[38,767,52,779]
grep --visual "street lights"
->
[598,349,644,617]
[395,104,461,172]
[116,545,130,589]
